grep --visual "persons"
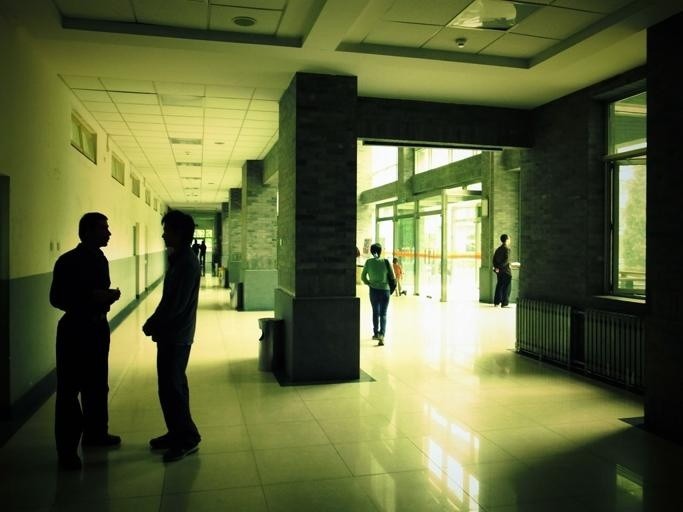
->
[44,212,122,471]
[197,241,207,264]
[139,208,204,461]
[390,258,402,297]
[491,234,513,308]
[360,242,396,346]
[191,240,200,256]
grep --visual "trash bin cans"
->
[219,267,226,288]
[258,318,285,372]
[215,262,219,276]
[229,282,242,309]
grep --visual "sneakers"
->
[373,334,384,345]
[150,432,201,462]
[81,434,121,447]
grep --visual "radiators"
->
[515,296,644,391]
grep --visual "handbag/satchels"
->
[385,260,396,294]
